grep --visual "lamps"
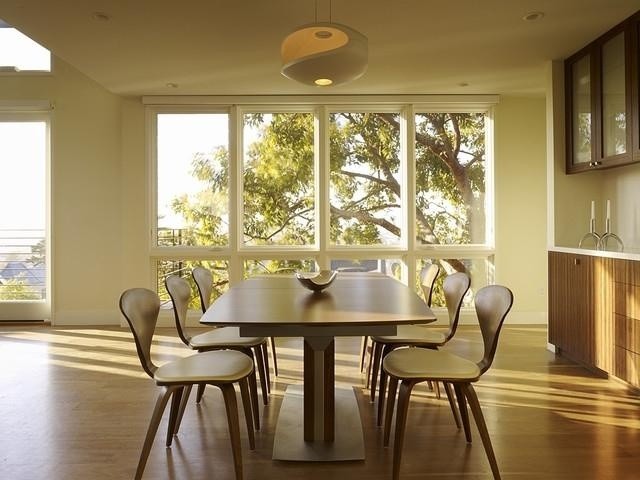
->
[278,0,369,89]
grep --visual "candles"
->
[591,199,595,221]
[606,199,611,221]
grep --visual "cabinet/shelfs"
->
[548,251,640,391]
[564,9,640,175]
